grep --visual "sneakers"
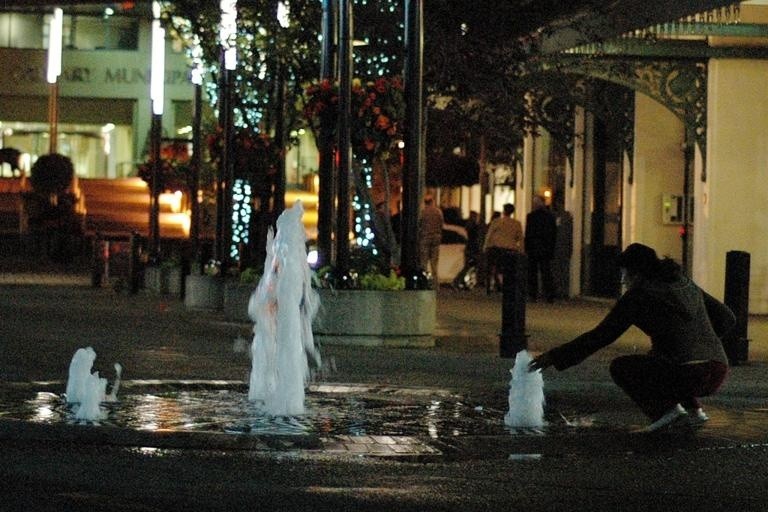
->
[626,401,710,438]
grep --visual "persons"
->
[526,239,741,433]
[377,193,571,303]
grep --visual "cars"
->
[424,223,481,288]
